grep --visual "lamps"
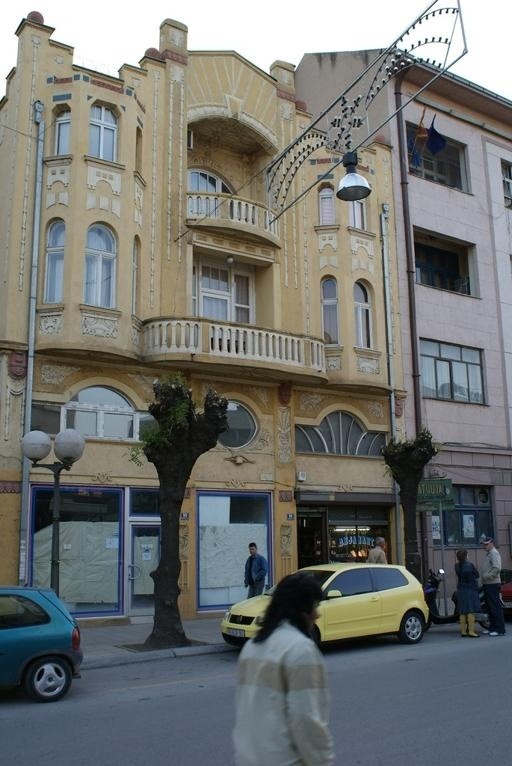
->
[298,471,306,482]
[226,255,233,263]
[336,150,371,201]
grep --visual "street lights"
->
[22,426,85,598]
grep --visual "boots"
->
[468,613,480,636]
[460,615,468,637]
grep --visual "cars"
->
[221,563,430,653]
[500,580,512,616]
[2,588,83,703]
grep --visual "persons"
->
[477,536,509,638]
[243,541,271,598]
[453,549,482,638]
[369,536,390,568]
[233,572,340,766]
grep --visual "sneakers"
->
[482,630,503,636]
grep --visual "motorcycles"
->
[421,563,492,630]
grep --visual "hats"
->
[481,537,498,546]
